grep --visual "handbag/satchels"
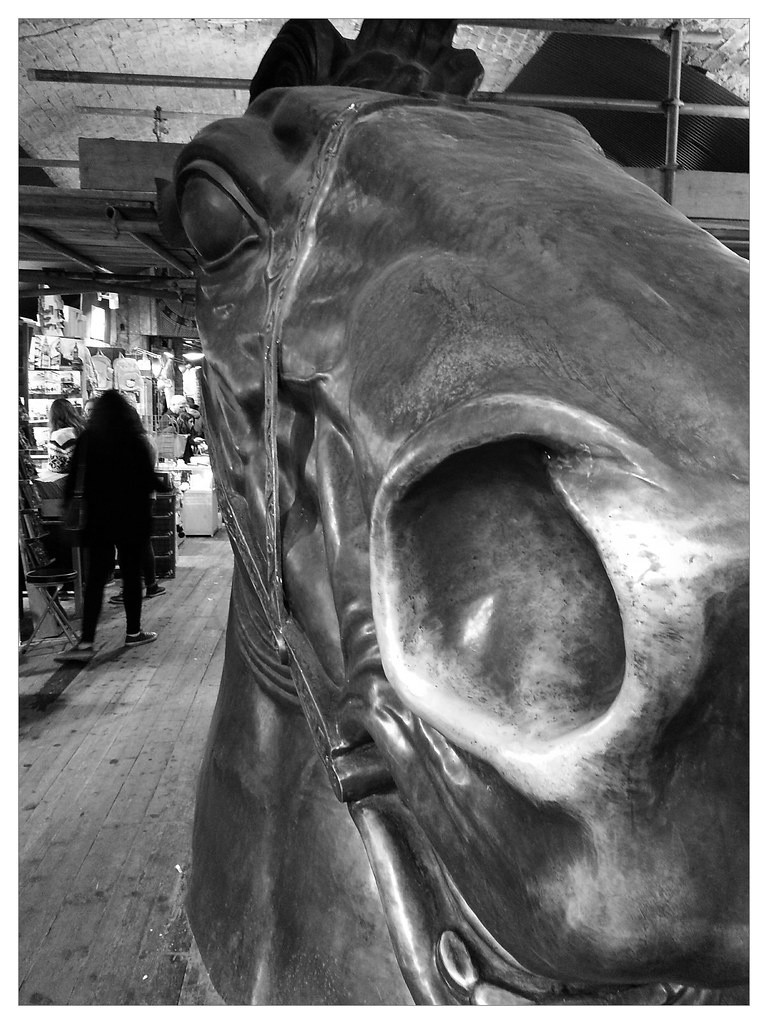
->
[63,489,88,543]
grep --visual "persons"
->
[110,405,166,605]
[82,397,98,421]
[161,411,194,464]
[159,394,187,432]
[53,389,158,665]
[186,397,195,408]
[184,404,204,438]
[48,398,116,597]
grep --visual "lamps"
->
[182,345,204,361]
[163,338,174,359]
[97,291,119,310]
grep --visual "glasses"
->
[81,408,96,415]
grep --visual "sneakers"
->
[68,642,94,652]
[147,584,166,596]
[124,630,157,645]
[110,590,125,604]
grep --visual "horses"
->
[140,80,749,1007]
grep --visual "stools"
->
[21,566,79,653]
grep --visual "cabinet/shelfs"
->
[155,454,224,536]
[140,472,175,579]
[19,316,84,454]
[19,468,124,592]
